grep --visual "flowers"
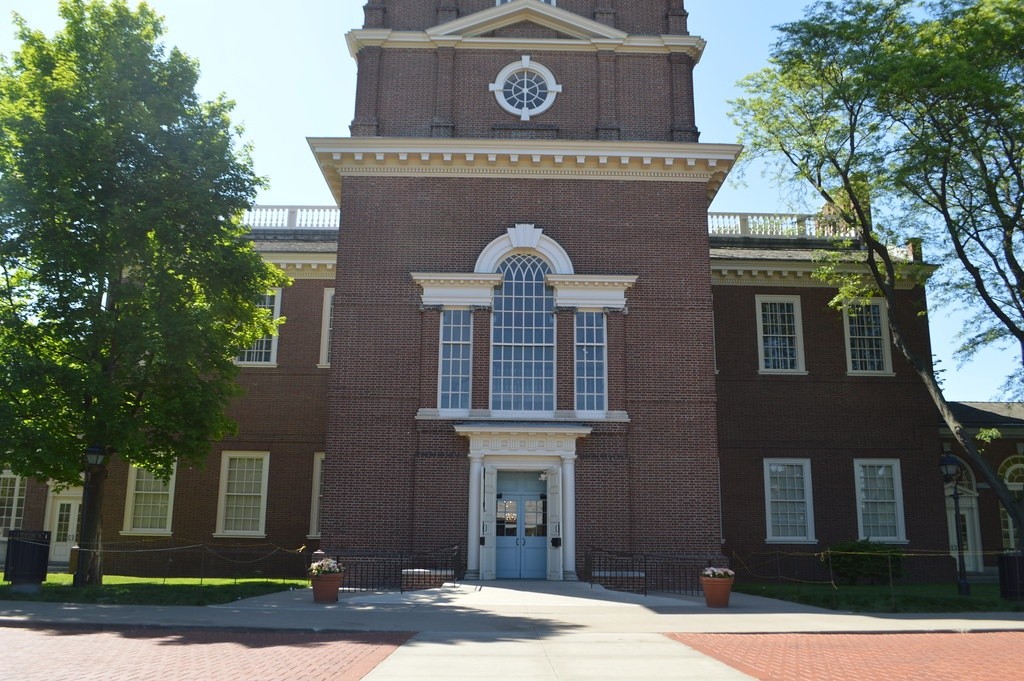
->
[700,567,735,578]
[308,558,345,577]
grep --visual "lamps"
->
[939,452,960,483]
[85,441,106,474]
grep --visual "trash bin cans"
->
[997,549,1024,600]
[3,528,51,582]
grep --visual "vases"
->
[310,574,343,604]
[701,577,734,608]
